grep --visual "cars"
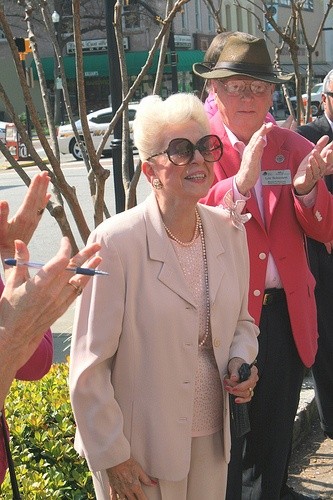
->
[56,104,139,161]
[285,82,324,117]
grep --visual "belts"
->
[262,287,287,305]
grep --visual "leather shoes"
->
[260,488,313,500]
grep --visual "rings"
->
[249,387,254,397]
[38,208,44,215]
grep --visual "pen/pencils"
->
[3,259,110,279]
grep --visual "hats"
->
[192,36,295,84]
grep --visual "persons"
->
[293,69,333,440]
[0,171,104,500]
[196,36,333,500]
[269,87,279,118]
[202,32,296,131]
[68,91,261,500]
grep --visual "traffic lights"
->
[14,36,32,55]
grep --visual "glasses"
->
[325,92,333,98]
[212,77,271,96]
[146,135,224,166]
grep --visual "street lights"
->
[51,11,61,125]
